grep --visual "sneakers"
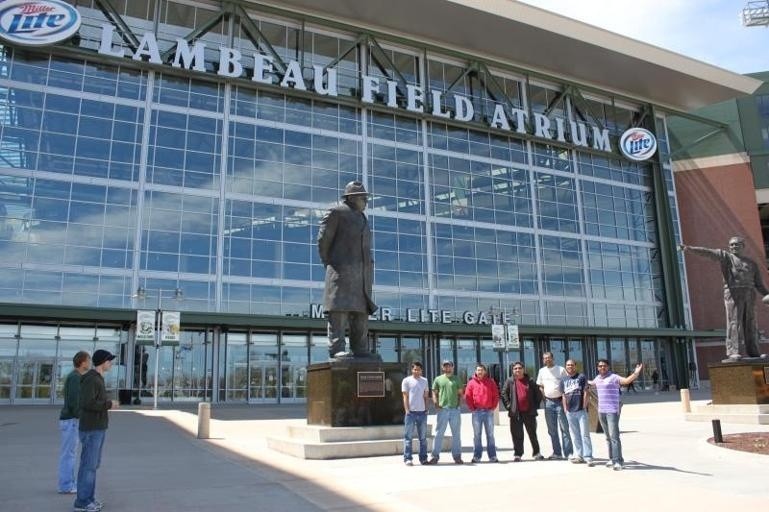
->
[58,489,77,494]
[75,500,104,512]
[407,455,624,470]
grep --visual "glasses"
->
[513,368,522,370]
[597,365,607,368]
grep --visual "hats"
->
[93,350,115,367]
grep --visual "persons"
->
[501,362,545,460]
[627,371,637,393]
[56,351,90,494]
[430,360,464,464]
[317,180,378,358]
[401,362,429,466]
[73,350,119,512]
[679,236,769,360]
[536,351,575,461]
[651,371,659,394]
[559,359,596,466]
[465,365,500,463]
[587,359,643,471]
